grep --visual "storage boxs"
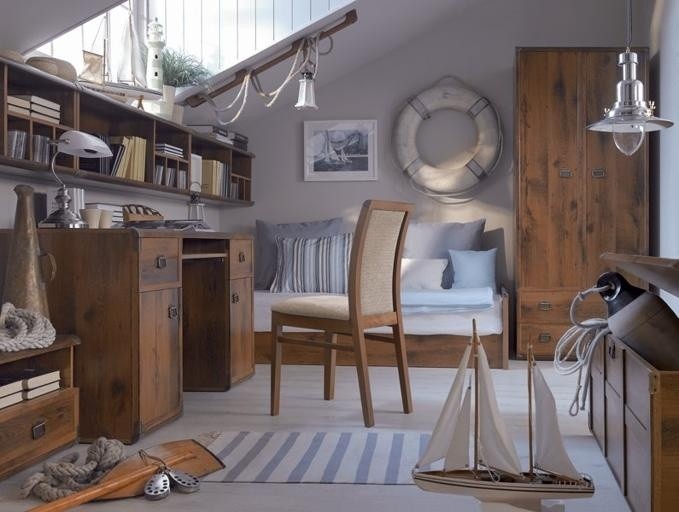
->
[587,250,679,512]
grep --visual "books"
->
[7,95,256,201]
[85,202,123,229]
[1,368,63,410]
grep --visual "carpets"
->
[196,429,438,484]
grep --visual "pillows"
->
[253,218,500,295]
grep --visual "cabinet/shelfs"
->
[0,334,82,482]
[0,56,256,207]
[512,46,650,359]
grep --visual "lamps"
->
[37,130,114,228]
[294,37,319,113]
[584,0,674,156]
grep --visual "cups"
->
[100,208,114,229]
[87,209,101,229]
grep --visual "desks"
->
[0,228,256,444]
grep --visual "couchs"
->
[253,284,509,370]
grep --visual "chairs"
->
[271,200,416,427]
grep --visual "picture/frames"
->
[303,119,378,182]
